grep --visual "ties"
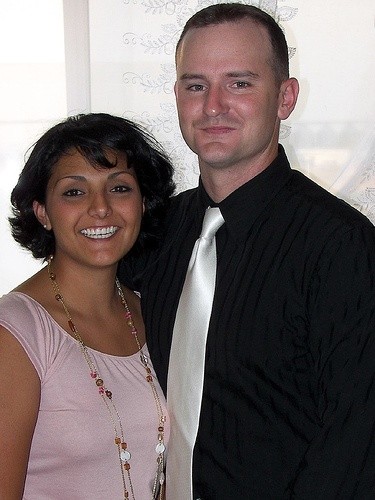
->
[164,205,226,500]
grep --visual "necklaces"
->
[46,257,166,500]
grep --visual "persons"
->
[0,112,176,500]
[116,3,375,500]
